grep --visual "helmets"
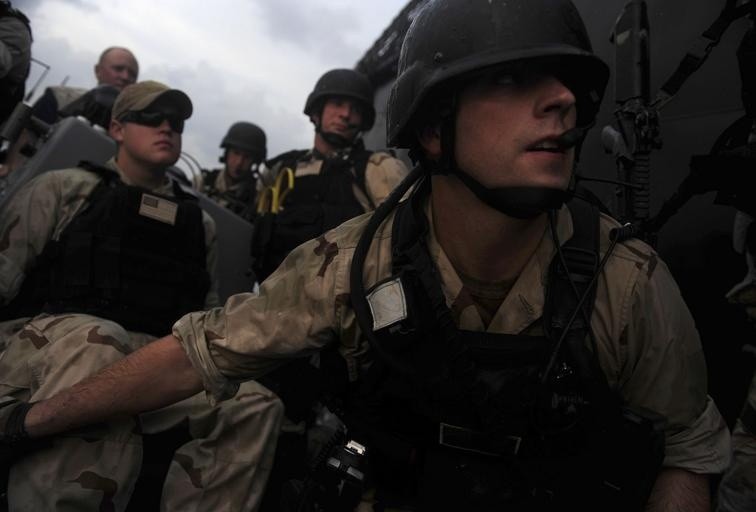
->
[220,123,266,162]
[386,0,610,151]
[304,69,375,131]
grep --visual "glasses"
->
[122,109,184,135]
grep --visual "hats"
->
[112,82,192,123]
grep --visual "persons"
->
[197,121,265,216]
[1,1,32,137]
[250,68,410,290]
[1,80,281,510]
[0,1,732,509]
[32,45,136,121]
